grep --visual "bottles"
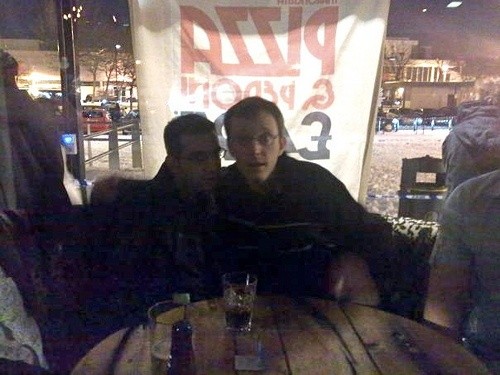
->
[167,322,197,375]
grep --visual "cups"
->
[148,303,200,375]
[223,273,258,334]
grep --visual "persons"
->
[79,96,417,336]
[422,169,500,375]
[442,98,500,199]
[0,50,65,375]
[391,116,453,132]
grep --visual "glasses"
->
[228,132,281,147]
[189,146,227,165]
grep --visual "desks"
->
[72,295,490,375]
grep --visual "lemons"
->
[156,307,183,325]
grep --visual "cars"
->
[81,94,138,133]
[377,106,459,132]
